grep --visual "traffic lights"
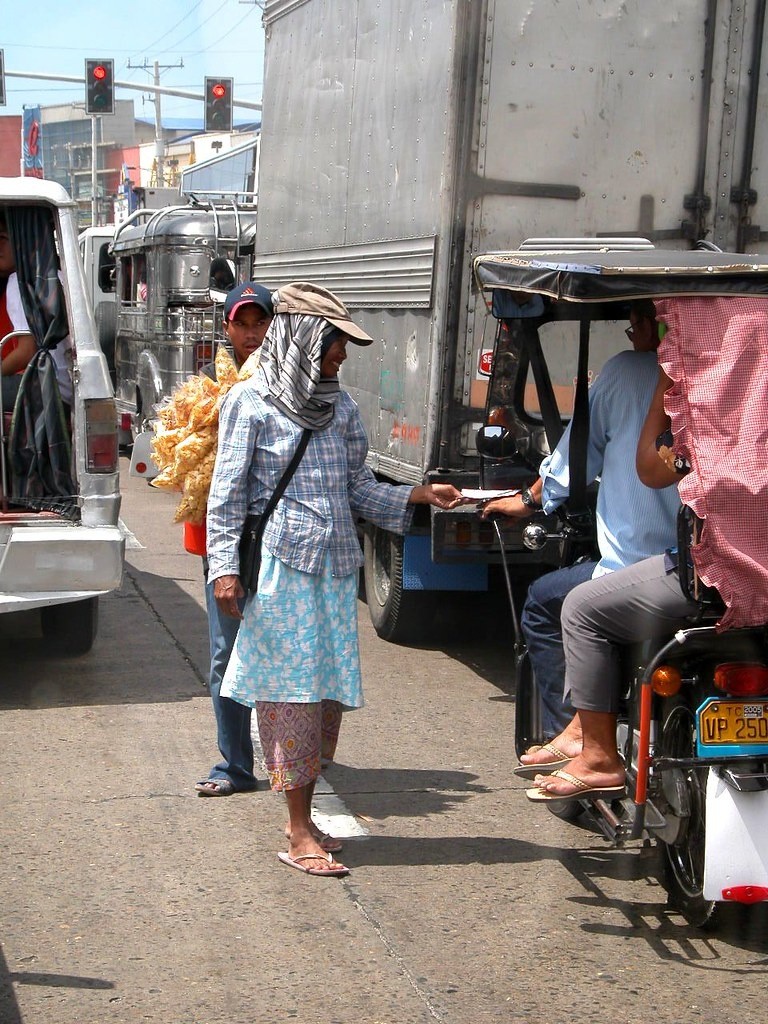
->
[86,58,115,115]
[204,76,234,134]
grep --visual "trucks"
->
[109,0,768,641]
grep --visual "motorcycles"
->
[473,238,768,929]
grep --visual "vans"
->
[0,178,124,655]
[77,224,136,390]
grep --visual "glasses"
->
[624,318,650,342]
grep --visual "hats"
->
[274,282,374,347]
[221,282,274,323]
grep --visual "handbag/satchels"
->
[239,513,265,592]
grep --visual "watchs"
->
[521,488,542,511]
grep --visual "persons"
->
[476,298,677,743]
[159,282,274,797]
[512,323,727,802]
[0,222,38,376]
[204,283,464,875]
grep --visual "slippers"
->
[195,778,235,796]
[513,744,576,781]
[526,769,626,802]
[278,852,349,876]
[285,830,342,851]
